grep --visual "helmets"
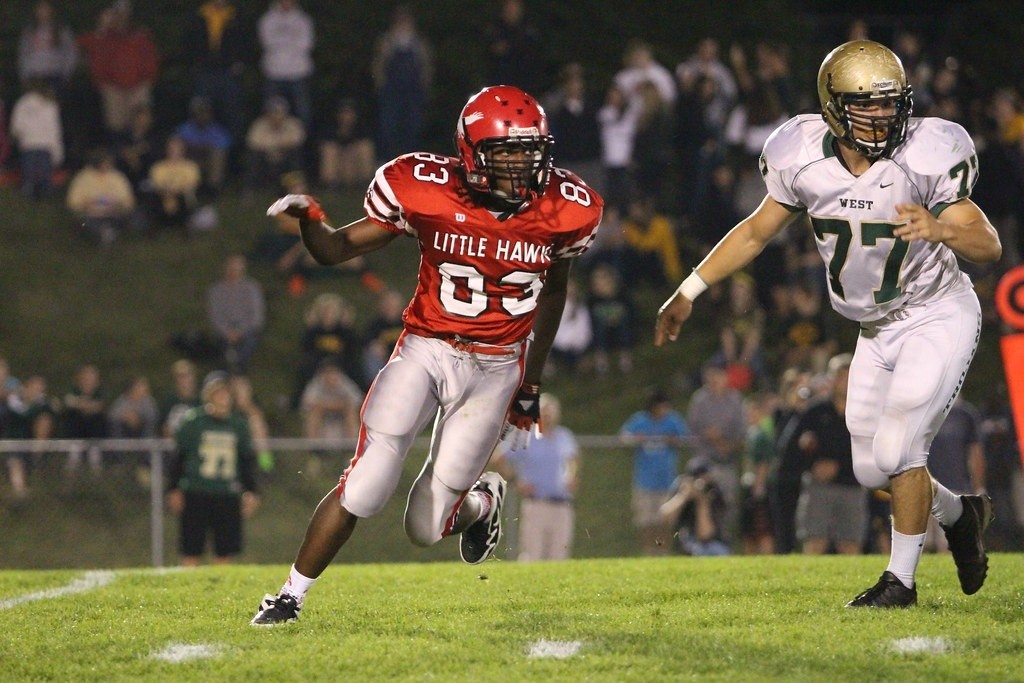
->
[817,40,914,159]
[453,84,556,214]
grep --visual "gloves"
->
[266,193,327,225]
[499,382,543,452]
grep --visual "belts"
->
[440,334,515,356]
[883,308,920,321]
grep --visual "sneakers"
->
[460,470,507,565]
[939,493,995,595]
[844,571,917,609]
[251,593,303,628]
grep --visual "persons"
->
[0,0,1024,555]
[165,378,258,568]
[248,85,604,626]
[655,39,1003,607]
[493,393,582,561]
[623,390,690,558]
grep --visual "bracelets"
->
[679,272,708,301]
